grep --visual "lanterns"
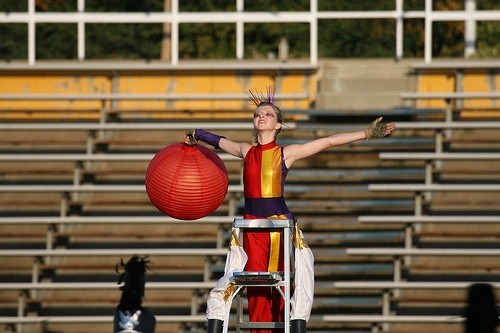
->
[145,133,229,221]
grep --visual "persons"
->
[185,101,395,333]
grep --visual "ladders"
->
[222,217,293,333]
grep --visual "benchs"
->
[0,64,500,333]
